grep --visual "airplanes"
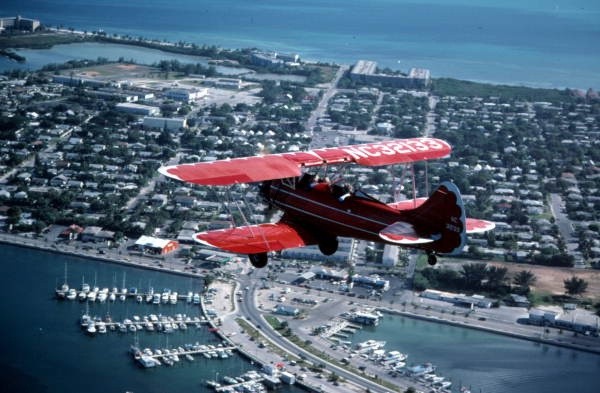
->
[157,138,495,268]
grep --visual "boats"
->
[104,315,115,331]
[170,292,177,304]
[159,316,173,333]
[417,374,445,387]
[146,290,154,302]
[174,346,194,362]
[98,288,108,302]
[361,350,384,361]
[350,339,386,354]
[129,287,137,295]
[163,349,178,361]
[143,316,154,331]
[130,345,155,367]
[109,287,117,301]
[117,322,127,333]
[235,377,245,382]
[153,294,161,304]
[119,288,127,301]
[207,344,228,358]
[143,348,153,355]
[88,286,99,301]
[206,379,220,388]
[377,350,408,366]
[123,318,136,332]
[67,289,76,299]
[200,344,218,357]
[162,293,168,304]
[150,314,162,331]
[384,361,406,371]
[167,316,179,329]
[87,321,96,333]
[193,344,212,358]
[186,292,193,302]
[134,316,142,331]
[96,317,106,334]
[193,294,201,305]
[176,314,187,330]
[78,284,90,300]
[156,349,174,366]
[224,376,238,384]
[194,316,202,328]
[136,295,143,302]
[430,382,452,393]
[56,284,69,300]
[218,343,235,356]
[153,358,161,365]
[404,362,437,379]
[81,314,90,326]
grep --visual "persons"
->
[310,176,323,187]
[338,183,355,201]
